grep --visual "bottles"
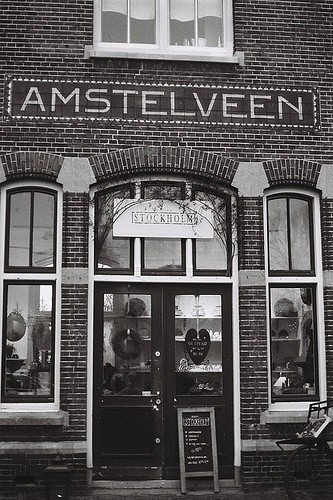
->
[29,347,51,389]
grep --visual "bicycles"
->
[276,398,333,499]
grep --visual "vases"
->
[191,37,207,47]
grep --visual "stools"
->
[44,465,73,500]
[15,483,35,500]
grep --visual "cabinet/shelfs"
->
[126,315,223,373]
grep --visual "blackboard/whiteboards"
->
[177,406,218,478]
